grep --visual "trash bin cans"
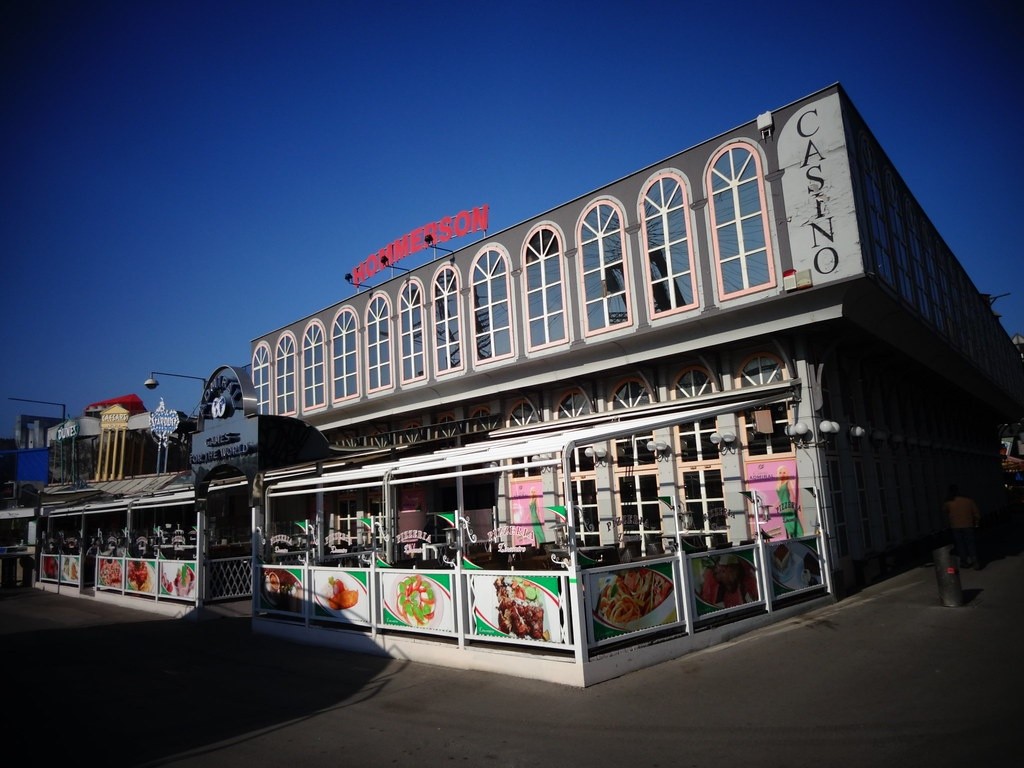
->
[932,544,964,608]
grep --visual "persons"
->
[943,488,983,571]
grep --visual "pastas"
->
[600,581,647,629]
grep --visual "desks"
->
[39,528,756,573]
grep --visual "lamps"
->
[747,431,755,443]
[532,454,552,471]
[486,462,501,475]
[646,441,667,458]
[851,425,867,453]
[585,448,607,464]
[617,447,625,461]
[424,234,454,252]
[380,256,412,270]
[785,422,810,450]
[144,372,206,391]
[709,432,735,452]
[818,420,840,447]
[680,441,687,456]
[344,273,372,288]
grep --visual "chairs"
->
[625,540,643,558]
[492,541,506,565]
[515,544,533,561]
[538,542,556,555]
[575,539,585,549]
[603,543,621,564]
[647,542,665,556]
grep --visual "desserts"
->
[771,543,820,587]
